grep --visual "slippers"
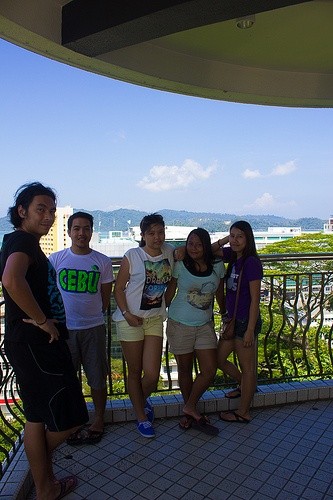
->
[181,415,219,435]
[221,410,252,423]
[53,474,78,500]
[224,387,258,399]
[79,423,104,440]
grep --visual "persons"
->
[112,215,185,437]
[0,182,89,500]
[211,221,263,423]
[47,212,113,445]
[164,228,228,430]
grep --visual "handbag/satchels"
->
[219,320,235,339]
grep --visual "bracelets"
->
[218,240,222,247]
[35,317,47,325]
[122,310,129,316]
[220,310,228,315]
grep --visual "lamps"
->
[236,14,255,29]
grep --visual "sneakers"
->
[143,400,154,424]
[136,420,155,438]
